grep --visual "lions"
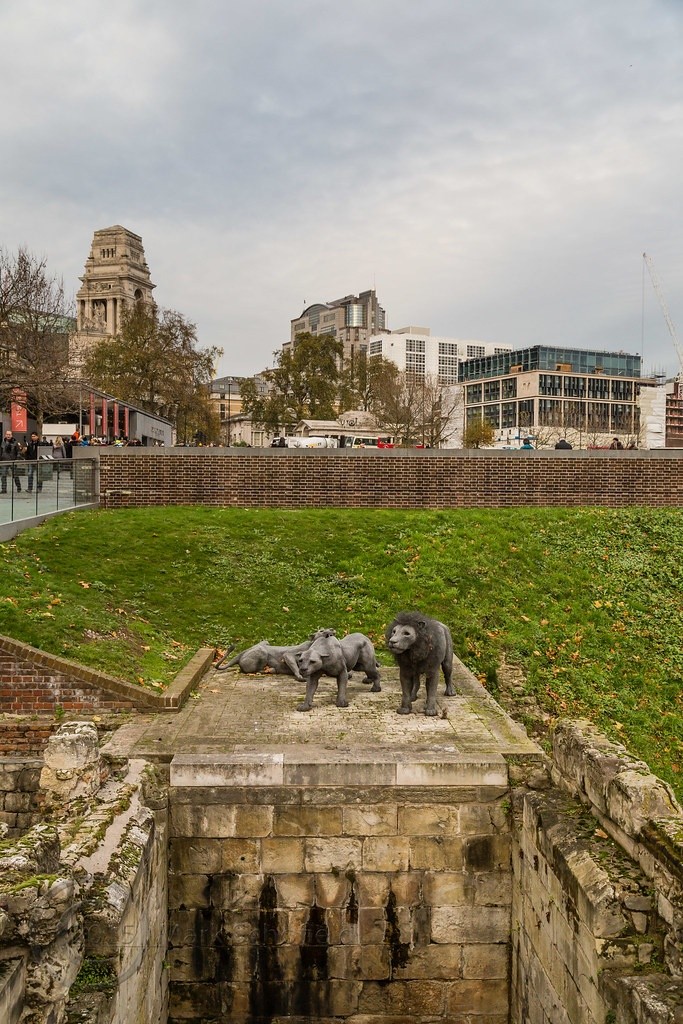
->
[295,632,381,712]
[214,628,336,682]
[385,613,456,716]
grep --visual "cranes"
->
[642,252,683,399]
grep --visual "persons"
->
[609,438,638,450]
[520,438,534,449]
[555,440,573,450]
[0,430,144,494]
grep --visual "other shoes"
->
[37,488,42,493]
[0,490,6,494]
[25,489,32,493]
[17,486,21,493]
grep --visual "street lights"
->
[228,380,233,448]
[519,401,528,445]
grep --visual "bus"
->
[272,437,337,448]
[345,436,402,448]
